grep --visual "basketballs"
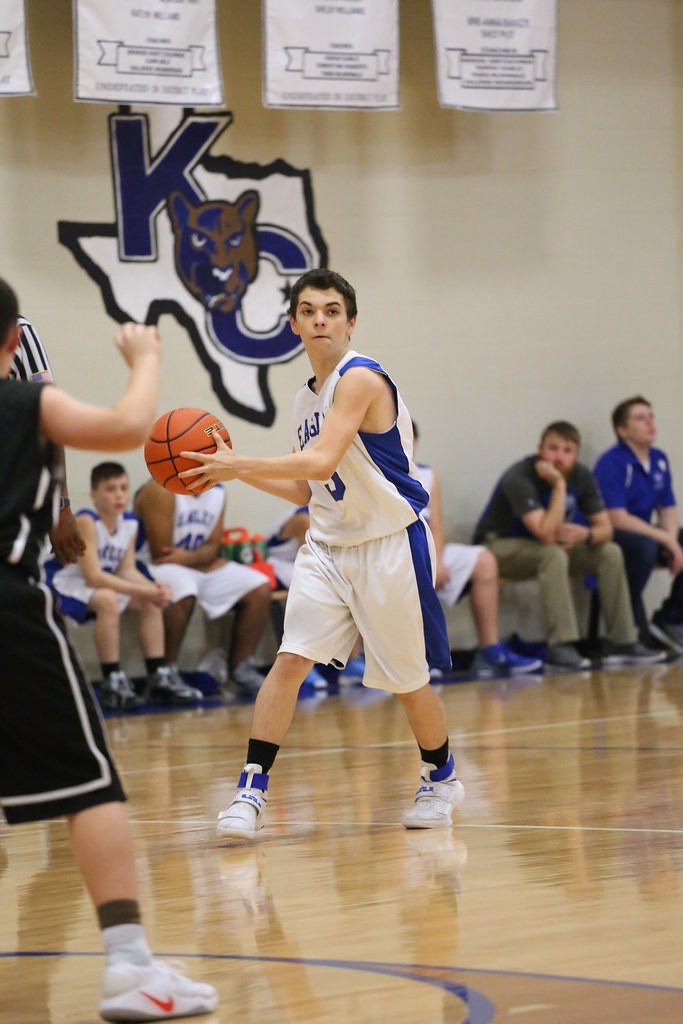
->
[144,407,233,497]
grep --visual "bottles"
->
[217,527,267,564]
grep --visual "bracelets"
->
[584,528,593,545]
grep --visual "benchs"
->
[60,566,601,700]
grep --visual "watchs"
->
[60,496,70,510]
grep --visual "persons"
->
[265,505,363,686]
[133,476,273,700]
[178,269,466,837]
[473,422,669,670]
[408,419,542,677]
[6,316,87,566]
[40,462,205,709]
[0,280,219,1024]
[574,396,683,657]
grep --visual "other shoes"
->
[646,619,683,658]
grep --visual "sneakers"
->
[472,643,546,674]
[145,666,207,705]
[400,759,464,829]
[95,956,220,1023]
[214,761,271,839]
[336,656,369,687]
[200,649,229,685]
[95,669,148,710]
[304,668,329,689]
[593,640,669,668]
[228,661,268,692]
[541,643,593,670]
